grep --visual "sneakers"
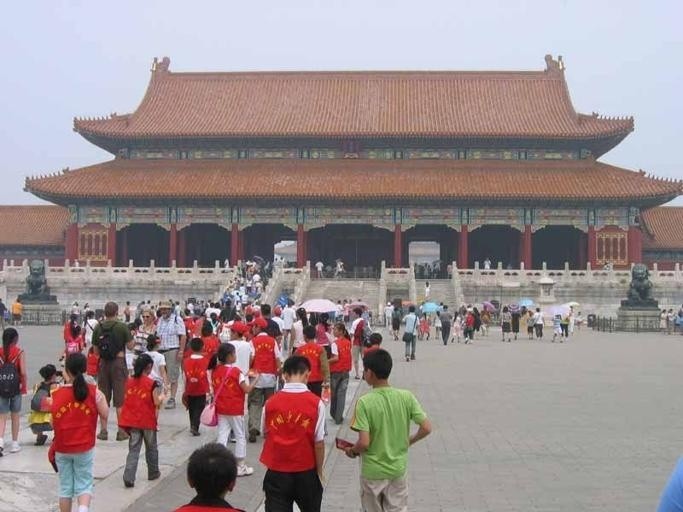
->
[147,471,160,480]
[237,464,254,477]
[249,431,256,442]
[116,431,129,441]
[10,443,21,453]
[165,398,175,408]
[190,427,200,436]
[97,430,108,441]
[0,440,6,457]
[122,473,134,487]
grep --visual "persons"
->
[603,262,611,270]
[316,259,346,279]
[1,298,23,324]
[659,305,683,332]
[385,301,584,361]
[483,258,491,275]
[658,460,683,511]
[223,258,272,308]
[414,260,451,279]
[424,281,431,296]
[1,328,27,456]
[29,294,433,511]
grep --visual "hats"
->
[225,320,247,334]
[158,299,172,309]
[249,318,267,329]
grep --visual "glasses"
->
[142,316,149,319]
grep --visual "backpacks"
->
[96,321,120,361]
[30,381,57,412]
[66,338,80,353]
[0,353,20,398]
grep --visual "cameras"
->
[55,370,63,376]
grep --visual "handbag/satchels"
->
[403,333,412,343]
[200,404,218,426]
